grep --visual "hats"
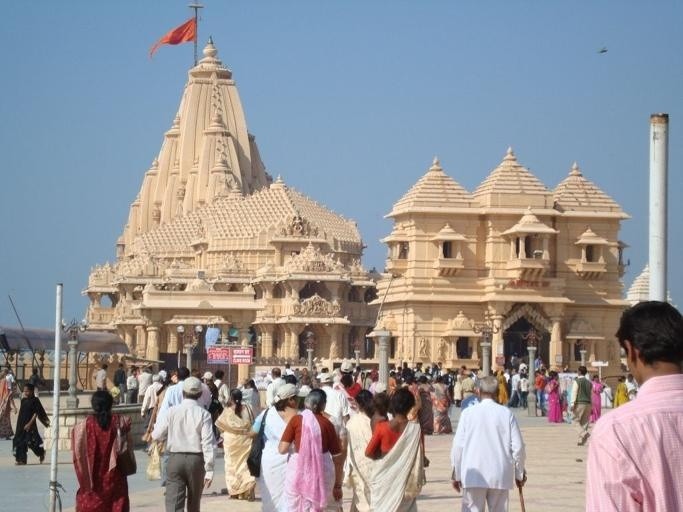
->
[340,360,354,374]
[462,370,473,377]
[271,383,300,404]
[201,371,213,380]
[182,376,203,395]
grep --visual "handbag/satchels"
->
[116,445,137,477]
[246,435,263,478]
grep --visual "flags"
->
[147,17,196,58]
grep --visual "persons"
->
[278,389,343,512]
[449,377,527,512]
[340,389,376,512]
[245,382,301,512]
[68,389,136,512]
[365,386,428,512]
[582,300,682,512]
[152,376,214,512]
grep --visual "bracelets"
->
[333,486,341,488]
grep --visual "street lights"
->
[518,327,545,417]
[61,316,92,410]
[174,324,203,375]
[303,329,316,372]
[574,336,591,369]
[478,325,493,378]
